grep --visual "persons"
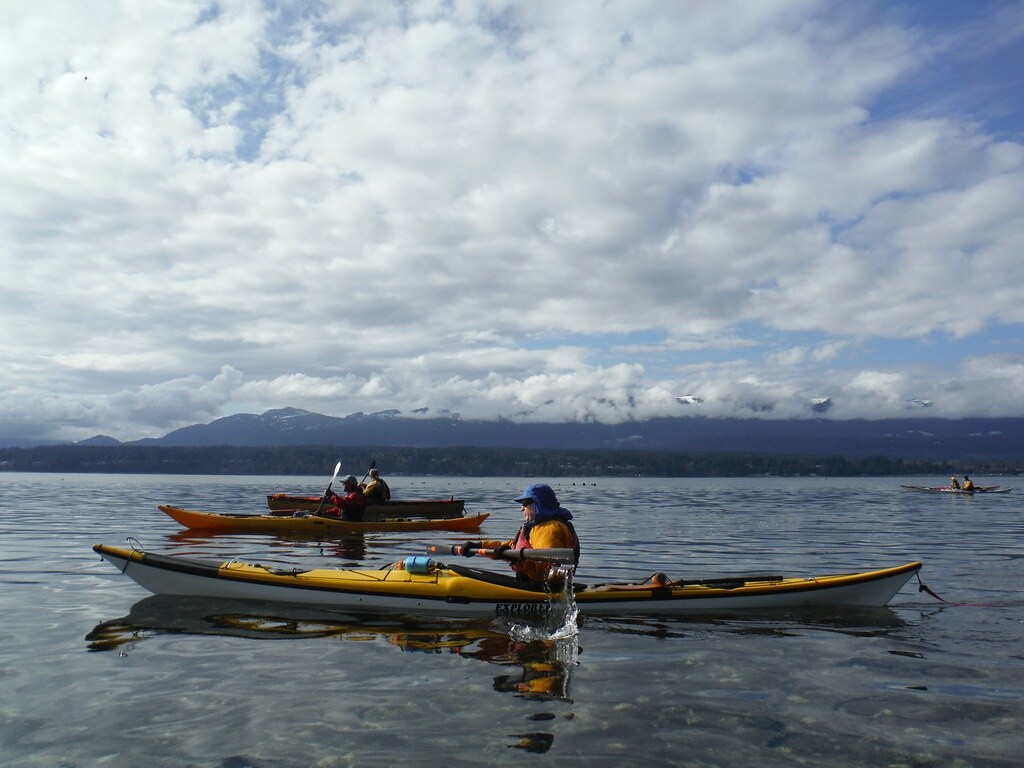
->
[961,475,974,490]
[457,483,580,592]
[950,477,959,490]
[358,469,391,501]
[314,475,367,521]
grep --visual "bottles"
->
[405,556,443,573]
[293,511,308,517]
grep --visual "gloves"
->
[324,489,334,498]
[314,512,322,515]
[493,544,518,562]
[461,541,482,558]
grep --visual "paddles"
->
[361,460,376,482]
[424,541,577,566]
[602,574,785,593]
[316,461,342,512]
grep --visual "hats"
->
[339,475,358,486]
[369,469,378,476]
[513,483,573,524]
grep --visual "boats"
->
[900,484,1012,495]
[267,493,466,520]
[158,504,490,534]
[90,537,923,619]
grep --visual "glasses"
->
[523,501,533,507]
